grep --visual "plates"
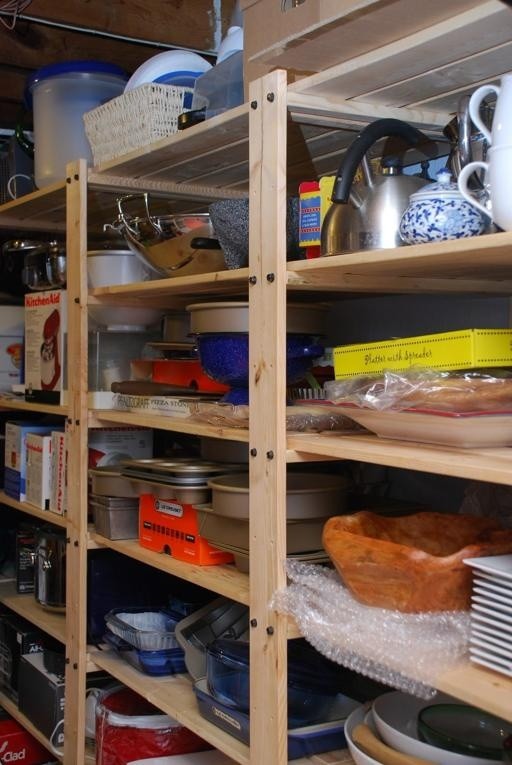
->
[463,546,512,687]
[345,685,511,765]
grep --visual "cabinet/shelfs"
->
[262,0,512,765]
[0,163,216,765]
[72,77,453,765]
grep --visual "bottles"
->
[11,522,69,615]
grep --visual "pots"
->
[101,192,230,280]
[0,236,68,290]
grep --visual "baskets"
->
[80,83,196,165]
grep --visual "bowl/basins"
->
[200,638,342,727]
[83,294,332,400]
[319,510,511,611]
[87,250,164,289]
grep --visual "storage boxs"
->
[24,290,68,406]
[18,652,115,747]
[333,328,511,382]
[0,615,59,707]
[28,433,51,511]
[0,306,24,397]
[3,420,63,502]
[50,430,153,514]
[238,0,486,104]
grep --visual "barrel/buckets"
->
[91,687,208,764]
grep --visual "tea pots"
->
[320,70,512,255]
